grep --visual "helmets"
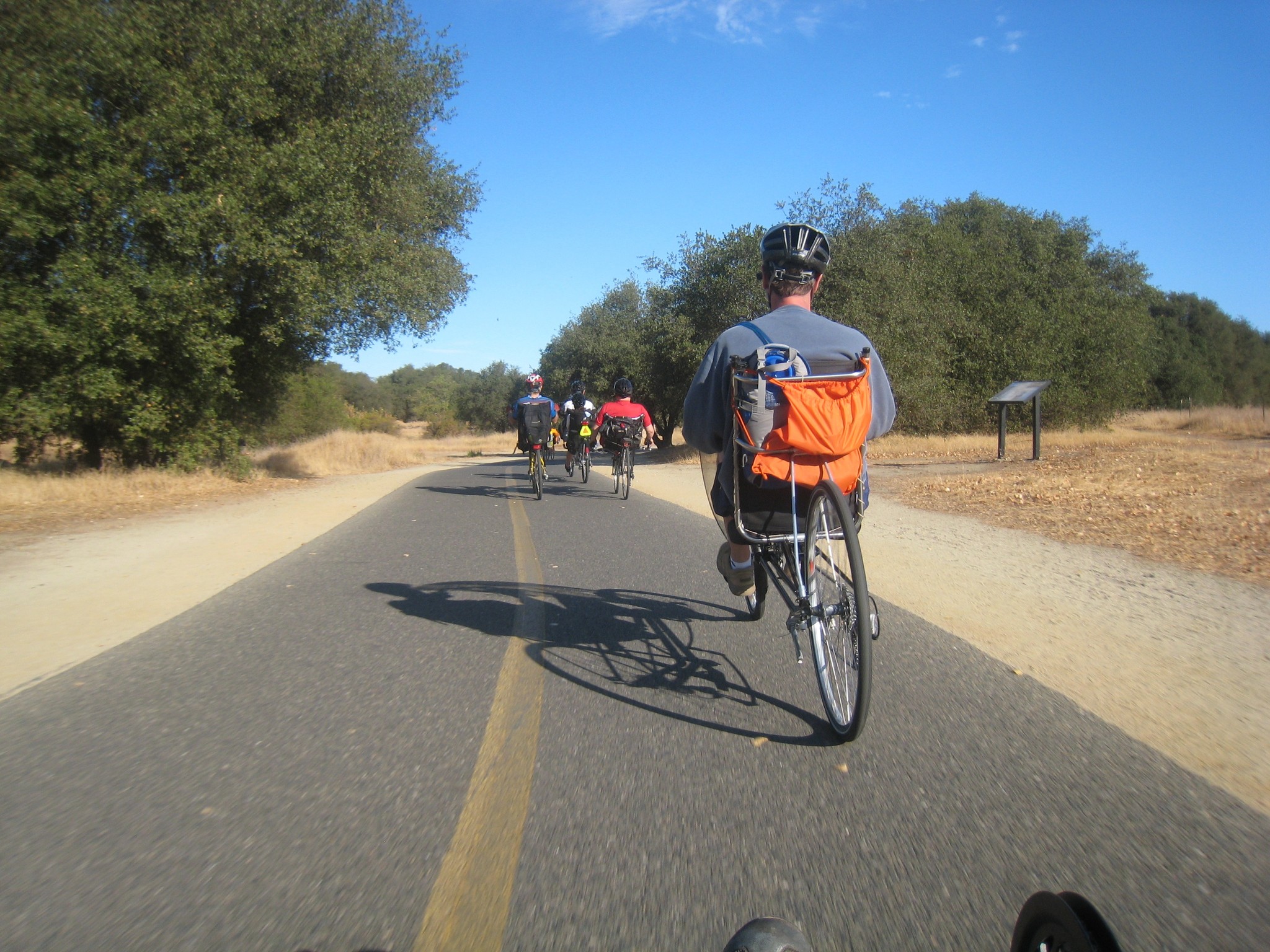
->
[571,380,586,391]
[759,222,832,271]
[526,374,543,389]
[613,378,632,396]
[572,392,585,407]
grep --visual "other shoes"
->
[516,443,528,451]
[543,443,549,450]
[552,432,556,444]
[565,463,571,472]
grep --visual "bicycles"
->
[700,344,880,742]
[546,408,597,484]
[589,413,651,499]
[511,401,556,499]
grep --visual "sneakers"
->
[717,540,756,595]
[784,558,802,585]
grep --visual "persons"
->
[683,222,896,598]
[512,374,656,470]
